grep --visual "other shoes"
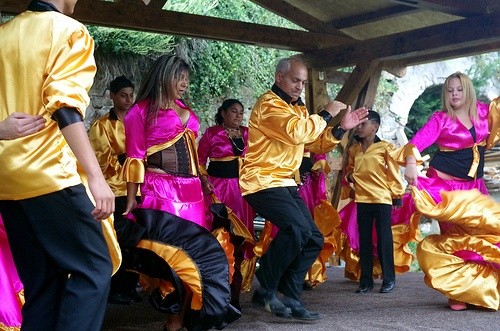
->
[109,292,132,305]
[448,299,467,310]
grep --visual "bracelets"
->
[406,163,417,164]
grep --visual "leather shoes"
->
[251,291,292,314]
[276,298,320,320]
[355,283,373,293]
[379,280,394,292]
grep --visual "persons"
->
[387,72,500,311]
[0,113,48,331]
[236,57,369,320]
[106,54,246,331]
[0,0,115,331]
[87,73,425,305]
